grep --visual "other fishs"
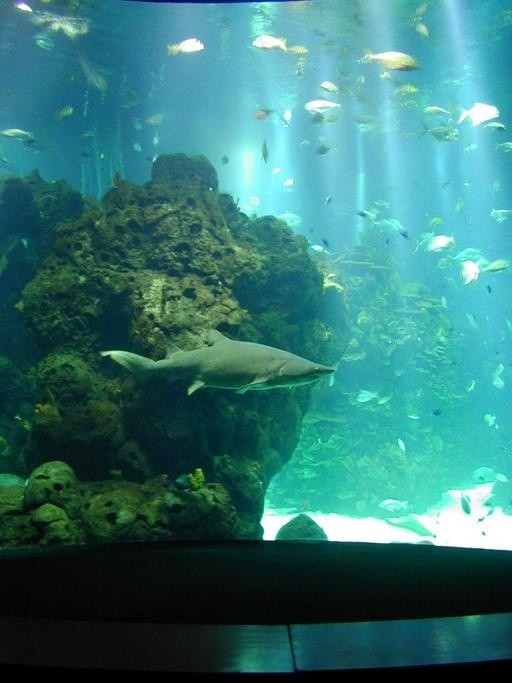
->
[332,294,512,549]
[0,0,512,294]
